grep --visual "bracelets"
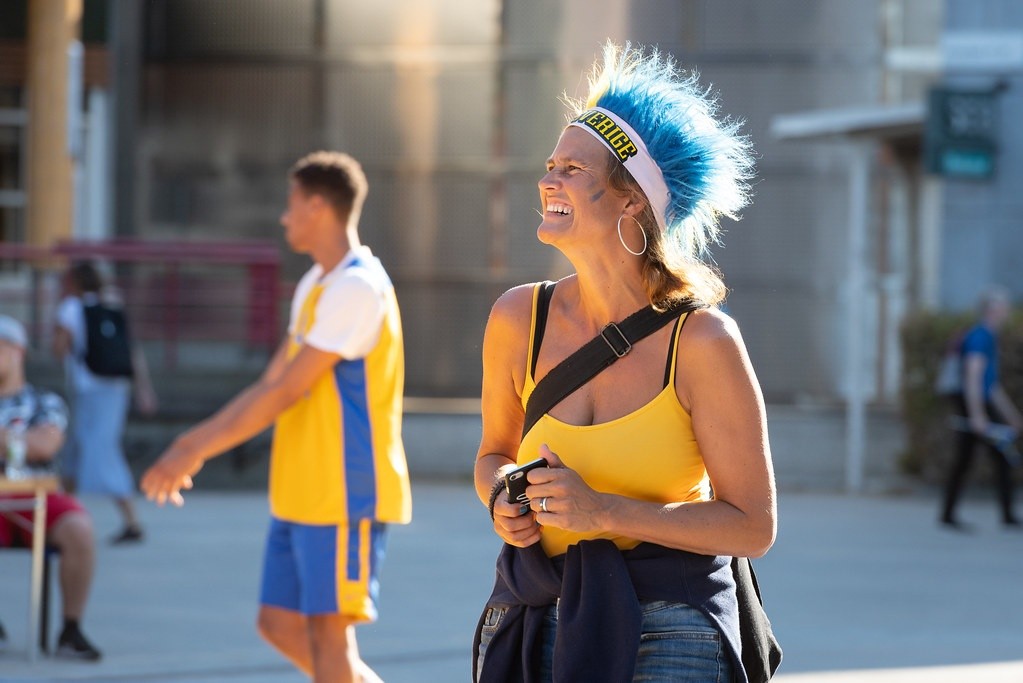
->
[488,480,506,522]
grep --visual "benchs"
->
[1,233,290,362]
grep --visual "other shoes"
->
[1002,514,1019,526]
[111,528,144,547]
[938,509,962,526]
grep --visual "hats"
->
[0,315,29,349]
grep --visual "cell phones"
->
[505,458,548,516]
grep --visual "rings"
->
[540,497,548,512]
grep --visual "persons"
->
[49,256,148,546]
[940,292,1023,528]
[0,315,106,662]
[136,153,414,683]
[468,50,777,683]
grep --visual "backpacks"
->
[82,304,133,378]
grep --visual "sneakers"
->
[55,622,102,660]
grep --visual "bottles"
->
[4,418,29,478]
